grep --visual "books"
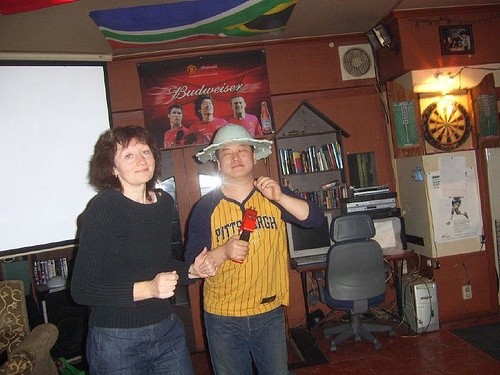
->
[279,138,344,175]
[281,176,347,210]
[33,253,69,284]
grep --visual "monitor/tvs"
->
[285,212,336,259]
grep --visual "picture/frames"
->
[438,24,475,55]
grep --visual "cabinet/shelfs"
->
[275,130,351,219]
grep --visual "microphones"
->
[232,209,257,264]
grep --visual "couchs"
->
[0,279,59,375]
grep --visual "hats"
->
[195,123,273,164]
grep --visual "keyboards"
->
[293,254,327,266]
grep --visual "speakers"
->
[30,283,89,373]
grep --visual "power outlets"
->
[462,285,472,300]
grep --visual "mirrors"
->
[155,176,189,308]
[198,172,223,198]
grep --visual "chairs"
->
[312,211,395,352]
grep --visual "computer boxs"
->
[401,277,440,334]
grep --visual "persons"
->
[184,122,324,375]
[163,94,263,147]
[70,126,220,375]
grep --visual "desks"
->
[290,251,414,330]
